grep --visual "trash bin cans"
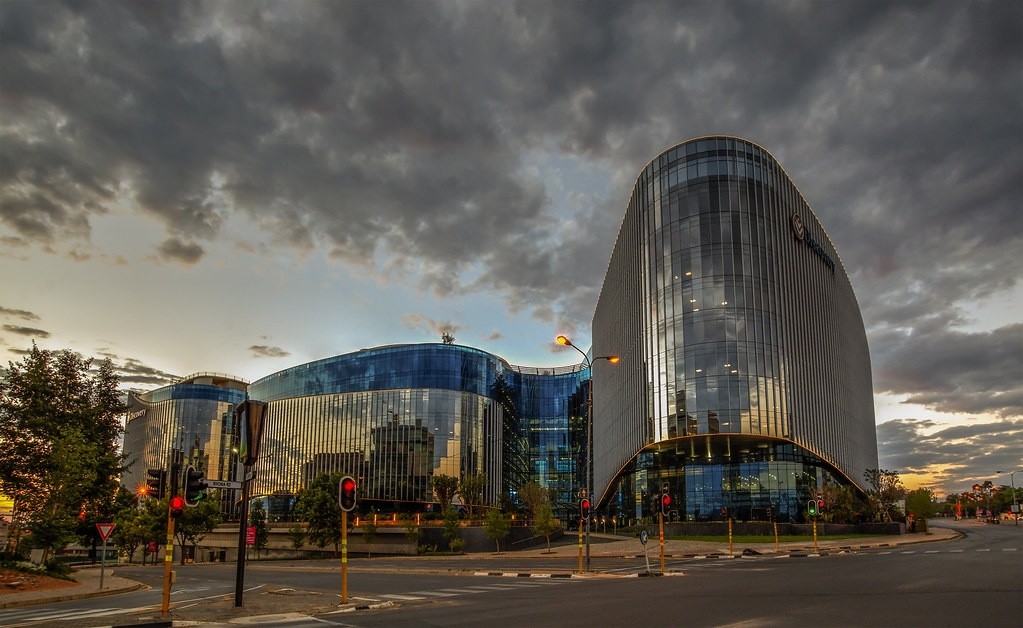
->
[205,550,226,562]
[914,519,926,532]
[685,513,695,522]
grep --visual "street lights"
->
[995,470,1023,525]
[555,335,622,573]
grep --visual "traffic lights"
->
[579,499,590,520]
[661,482,670,495]
[661,494,672,517]
[183,464,209,508]
[169,495,185,518]
[578,488,589,499]
[807,500,817,515]
[817,499,824,515]
[145,465,167,502]
[339,475,357,512]
